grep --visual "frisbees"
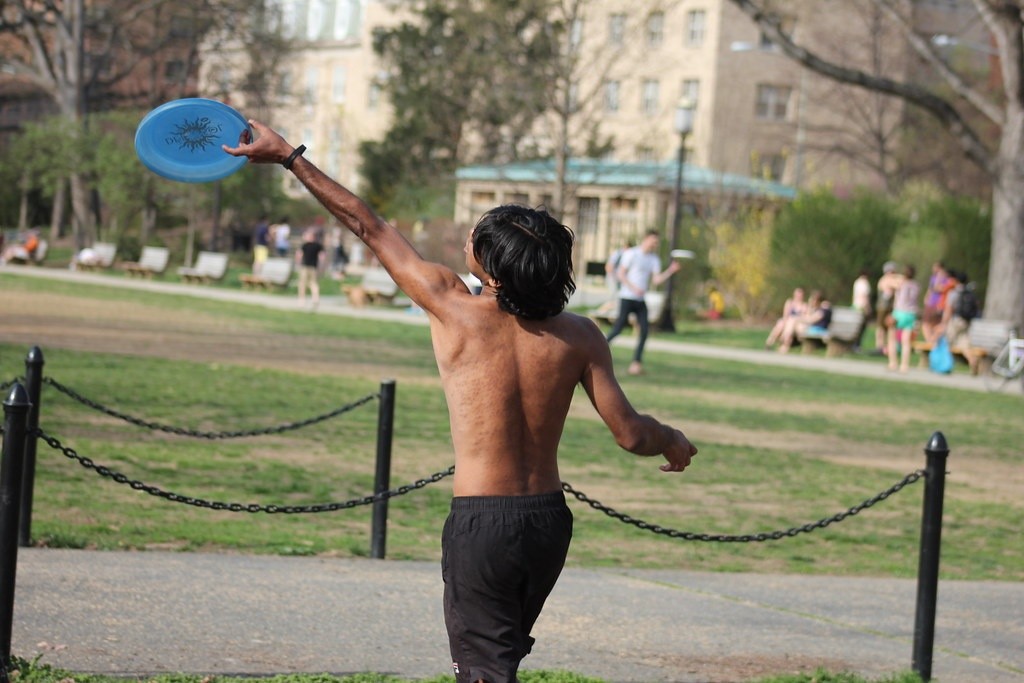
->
[671,249,697,259]
[134,97,253,183]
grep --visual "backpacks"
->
[953,288,976,320]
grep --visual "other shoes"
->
[627,363,646,374]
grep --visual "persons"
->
[222,118,700,683]
[694,285,726,320]
[764,260,981,377]
[227,213,683,376]
[0,229,41,269]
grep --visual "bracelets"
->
[282,145,308,171]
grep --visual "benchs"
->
[592,288,669,337]
[341,268,400,310]
[801,305,866,356]
[120,245,171,279]
[75,240,116,270]
[239,257,294,293]
[16,239,47,265]
[179,251,230,287]
[911,318,1018,366]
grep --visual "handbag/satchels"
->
[929,336,953,374]
[884,314,896,327]
